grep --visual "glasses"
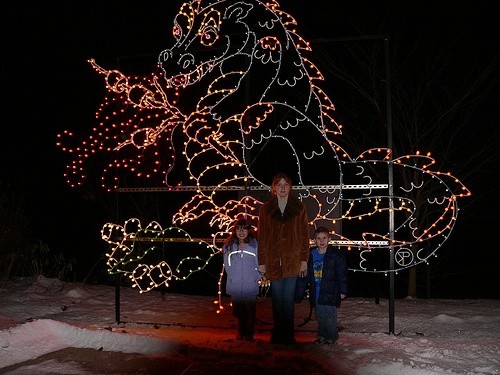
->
[236,229,248,232]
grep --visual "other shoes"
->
[312,336,326,347]
[320,339,337,348]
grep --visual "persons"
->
[222,219,263,342]
[303,226,350,347]
[257,173,313,343]
